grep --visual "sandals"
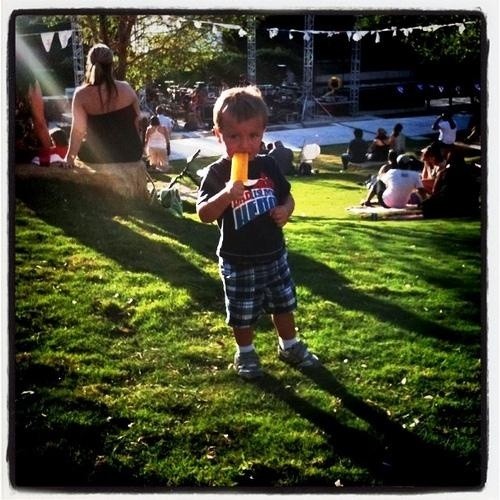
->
[235,352,262,380]
[276,342,319,371]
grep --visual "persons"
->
[389,123,410,155]
[143,115,171,168]
[338,127,366,173]
[359,143,458,210]
[154,104,174,135]
[267,140,294,175]
[47,127,68,163]
[195,87,320,382]
[431,109,458,145]
[15,50,69,167]
[59,42,146,169]
[365,127,390,161]
